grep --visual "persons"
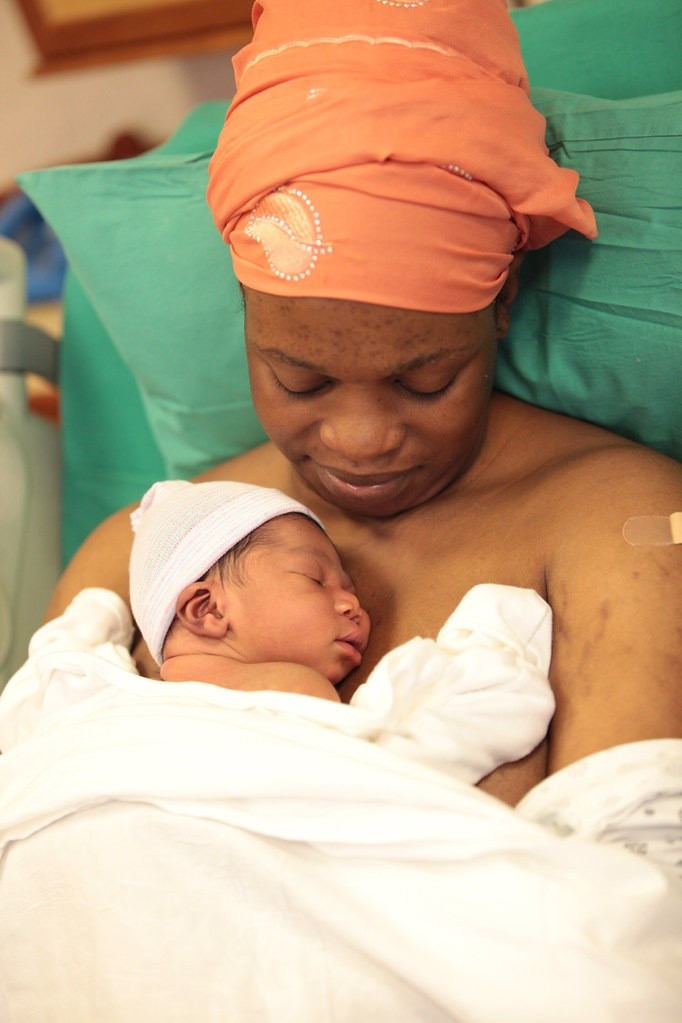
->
[46,0,682,809]
[129,481,371,710]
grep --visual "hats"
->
[128,480,329,667]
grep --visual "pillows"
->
[14,94,682,488]
